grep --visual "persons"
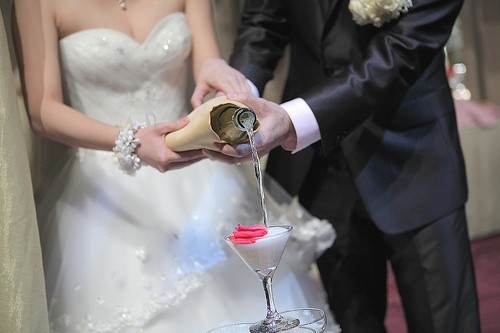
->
[220,0,479,333]
[12,1,337,333]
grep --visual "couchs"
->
[456,122,499,244]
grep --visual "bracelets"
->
[112,123,143,174]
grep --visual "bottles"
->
[211,103,257,144]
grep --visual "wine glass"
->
[223,226,300,333]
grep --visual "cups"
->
[207,308,327,333]
